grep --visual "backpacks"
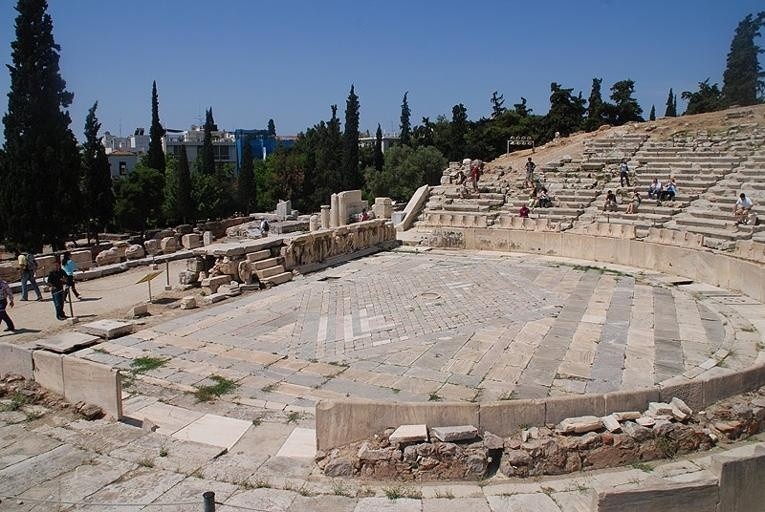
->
[20,255,36,271]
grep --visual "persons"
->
[733,193,753,225]
[0,246,80,332]
[519,157,550,218]
[456,163,479,198]
[262,221,269,237]
[361,208,369,222]
[603,158,677,214]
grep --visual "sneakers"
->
[35,296,42,301]
[59,314,66,320]
[19,298,27,301]
[76,296,82,299]
[4,326,13,331]
